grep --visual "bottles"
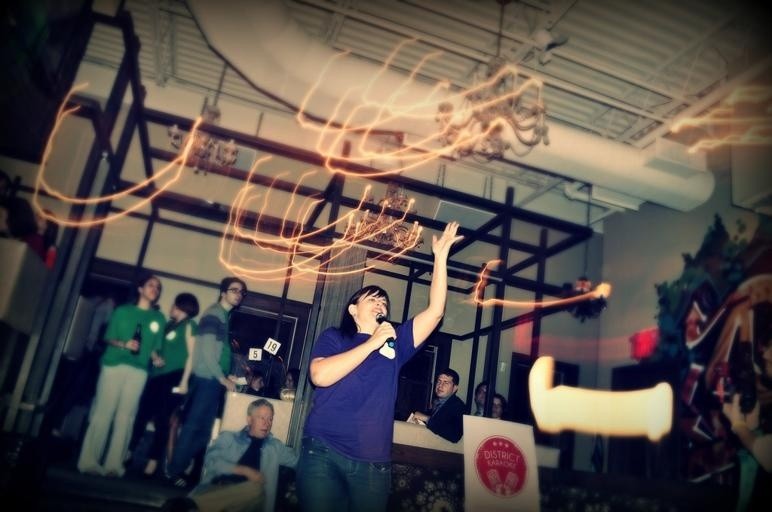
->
[130,323,142,354]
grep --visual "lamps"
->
[167,62,241,177]
[343,171,425,263]
[435,0,550,164]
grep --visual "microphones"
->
[375,312,395,348]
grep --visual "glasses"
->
[228,288,247,297]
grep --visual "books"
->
[406,411,427,426]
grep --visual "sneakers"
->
[81,462,192,490]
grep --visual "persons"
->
[489,392,508,422]
[37,277,117,442]
[121,292,200,478]
[228,335,302,401]
[297,219,466,509]
[164,277,245,491]
[475,381,489,417]
[161,397,300,512]
[74,272,167,475]
[411,366,467,443]
